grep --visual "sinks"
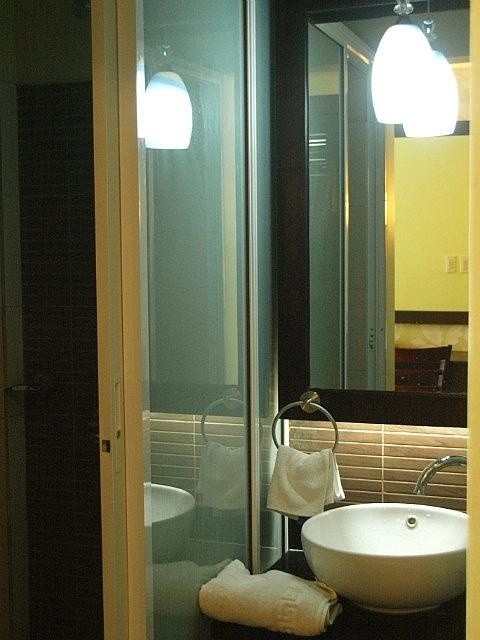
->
[144,481,196,545]
[300,502,470,613]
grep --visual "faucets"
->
[413,456,467,498]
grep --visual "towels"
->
[199,561,346,637]
[194,441,248,512]
[153,557,233,619]
[266,447,345,519]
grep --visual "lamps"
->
[145,1,191,148]
[400,0,458,138]
[371,0,435,125]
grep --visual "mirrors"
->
[268,2,470,429]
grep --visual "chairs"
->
[393,346,453,393]
